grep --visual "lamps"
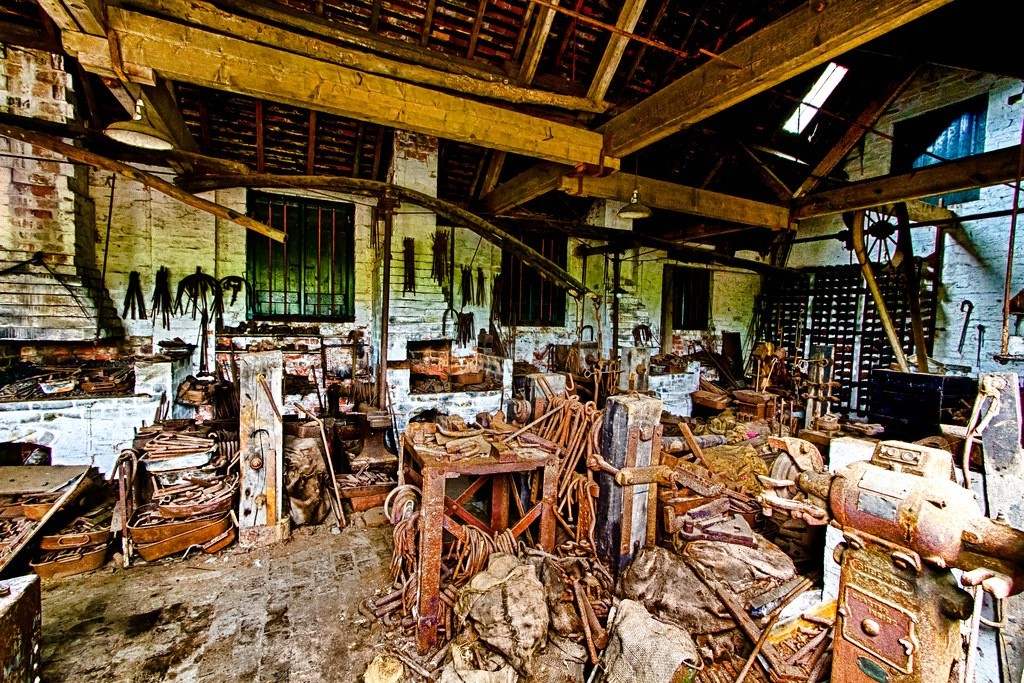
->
[617,151,653,220]
[102,82,177,150]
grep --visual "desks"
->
[399,431,556,652]
